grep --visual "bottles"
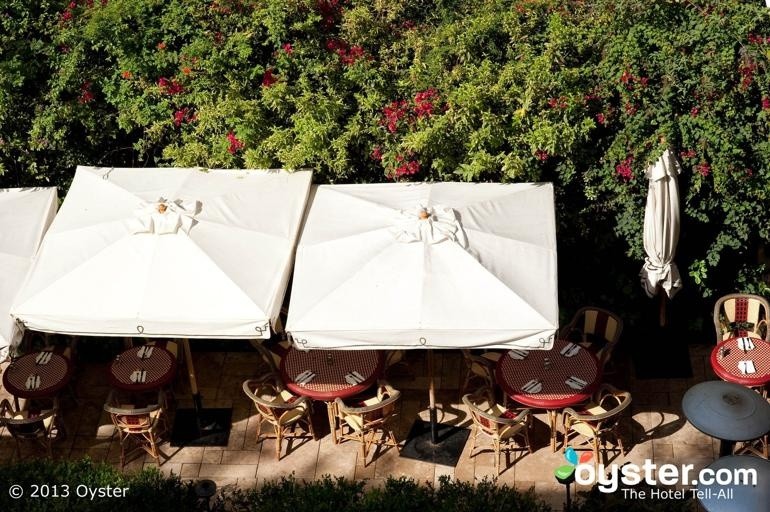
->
[327,353,334,365]
[115,355,120,370]
[544,357,550,371]
[719,347,724,359]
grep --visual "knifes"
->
[296,372,315,385]
[567,376,586,388]
[29,378,32,391]
[511,350,527,359]
[523,380,542,391]
[141,346,147,359]
[742,337,746,353]
[135,373,139,384]
[562,343,576,357]
[34,373,38,389]
[36,351,47,365]
[349,371,364,385]
[140,367,144,384]
[144,346,152,357]
[43,352,50,363]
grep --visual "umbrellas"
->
[639,138,683,353]
[9,164,313,427]
[284,180,558,444]
[0,184,58,370]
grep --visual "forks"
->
[747,338,751,348]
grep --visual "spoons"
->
[743,360,747,374]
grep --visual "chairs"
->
[0,293,770,477]
